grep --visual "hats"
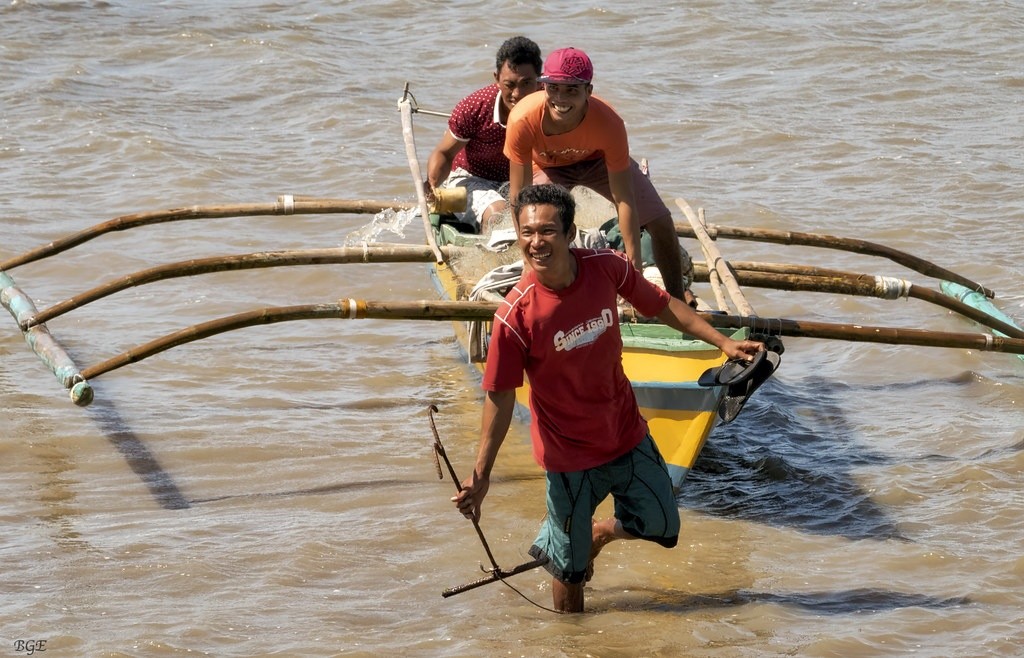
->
[535,47,593,84]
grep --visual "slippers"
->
[718,352,780,423]
[697,349,767,386]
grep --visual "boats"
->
[433,186,786,498]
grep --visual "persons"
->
[423,36,686,304]
[450,185,765,614]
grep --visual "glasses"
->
[546,84,587,95]
